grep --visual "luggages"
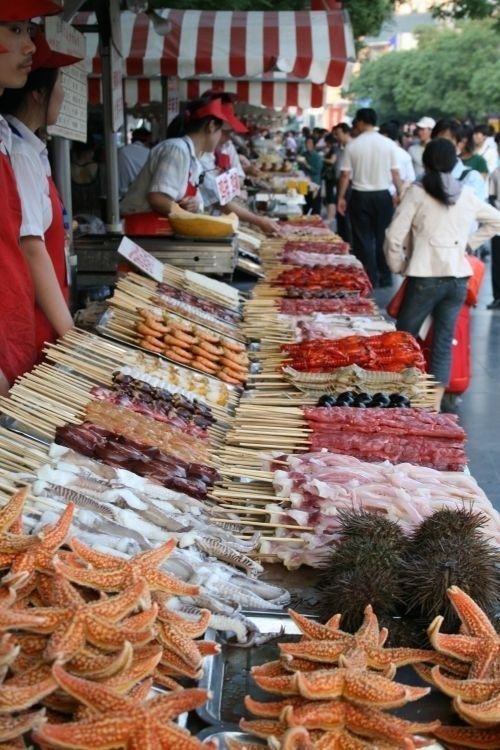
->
[416,300,473,407]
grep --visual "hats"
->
[415,115,437,129]
[0,0,62,22]
[32,32,83,75]
[196,96,251,135]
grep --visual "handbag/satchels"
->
[463,251,486,308]
[385,275,410,318]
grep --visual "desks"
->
[255,189,308,205]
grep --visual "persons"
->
[0,2,54,401]
[0,18,84,347]
[118,90,282,238]
[487,133,500,309]
[383,138,499,412]
[285,116,497,259]
[337,108,404,288]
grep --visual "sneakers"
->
[488,299,500,308]
